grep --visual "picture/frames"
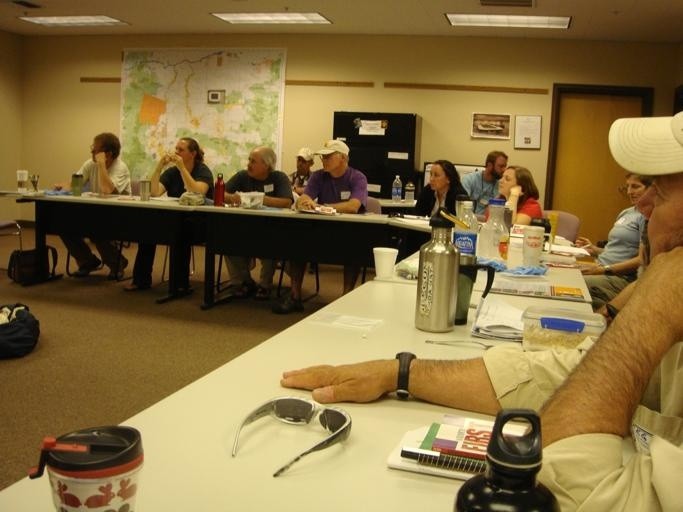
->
[470,112,511,139]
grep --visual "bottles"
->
[214,173,225,206]
[404,181,415,204]
[392,175,402,204]
[415,200,509,334]
[452,407,563,512]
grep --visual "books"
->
[385,413,529,482]
[550,286,584,302]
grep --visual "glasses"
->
[232,398,351,478]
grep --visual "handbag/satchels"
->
[7,249,48,283]
[0,303,39,359]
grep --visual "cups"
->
[139,180,152,201]
[372,247,399,279]
[241,192,264,208]
[522,225,553,268]
[26,423,146,512]
[72,174,83,198]
[17,171,28,193]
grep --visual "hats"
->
[313,140,350,156]
[296,148,314,162]
[178,191,214,205]
[609,111,682,176]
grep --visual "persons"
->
[573,168,652,326]
[288,147,315,197]
[122,137,214,296]
[279,111,683,512]
[460,151,508,223]
[224,146,294,300]
[414,160,470,218]
[485,166,543,226]
[54,132,132,280]
[272,139,368,314]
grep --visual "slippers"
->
[124,282,138,290]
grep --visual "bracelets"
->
[396,352,416,401]
[602,265,611,275]
[293,186,296,192]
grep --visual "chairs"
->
[0,180,382,303]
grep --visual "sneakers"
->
[108,258,127,281]
[74,258,101,277]
[233,280,255,297]
[271,297,304,314]
[255,287,271,299]
